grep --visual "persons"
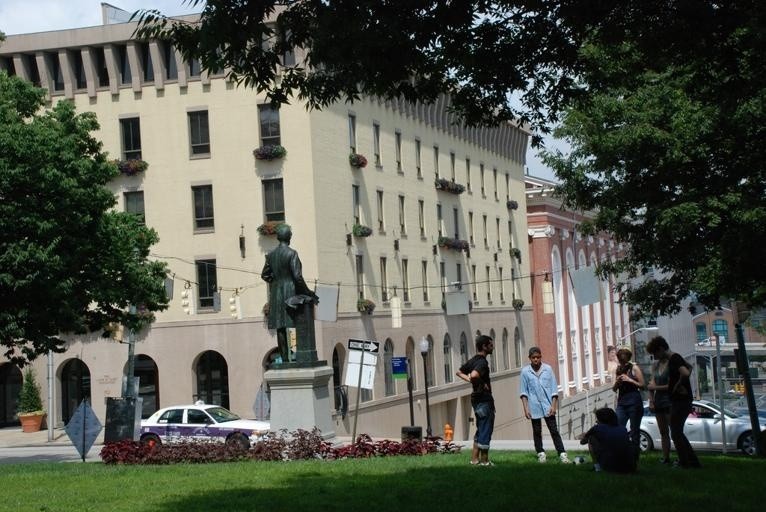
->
[520,347,571,463]
[260,225,320,363]
[580,407,638,475]
[646,336,703,468]
[456,335,497,465]
[647,358,681,465]
[612,348,646,462]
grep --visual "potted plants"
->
[14,367,45,432]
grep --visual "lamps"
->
[390,288,402,328]
[229,289,242,320]
[180,280,194,316]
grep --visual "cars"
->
[139,399,276,452]
[626,398,766,458]
[732,406,766,419]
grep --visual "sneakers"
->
[470,460,495,467]
[660,456,701,469]
[537,452,547,463]
[559,453,571,463]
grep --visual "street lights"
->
[418,333,437,446]
[614,325,660,344]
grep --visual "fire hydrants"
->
[443,423,453,443]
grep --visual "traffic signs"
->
[347,336,380,355]
